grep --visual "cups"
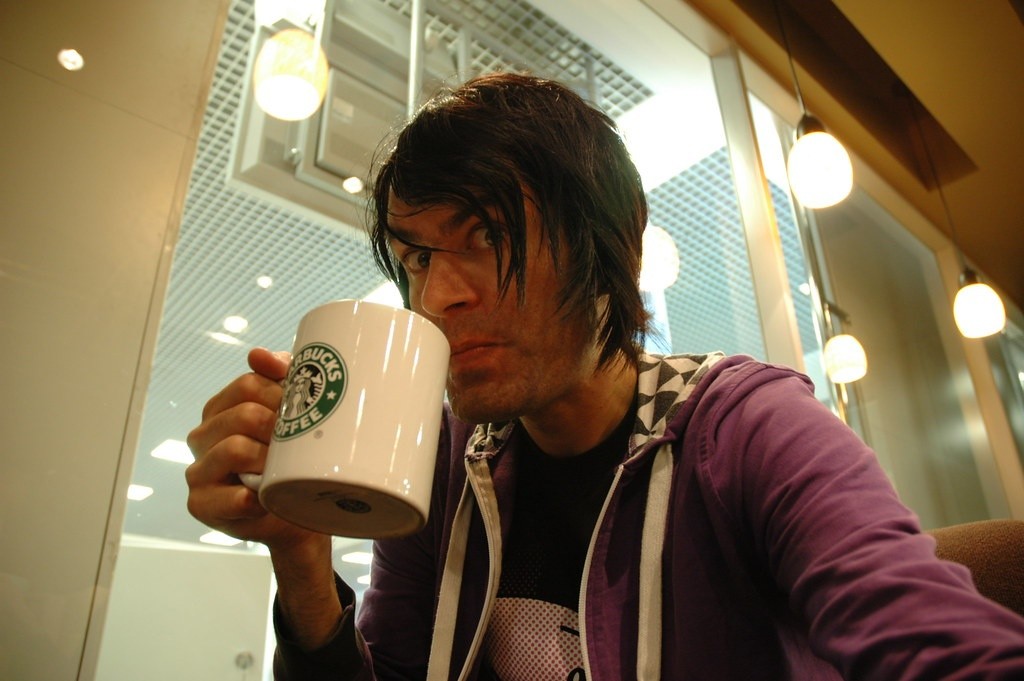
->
[237,301,450,539]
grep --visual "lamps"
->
[638,216,680,292]
[774,0,854,209]
[893,82,1006,339]
[253,29,330,121]
[823,334,868,385]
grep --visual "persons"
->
[184,70,1024,681]
